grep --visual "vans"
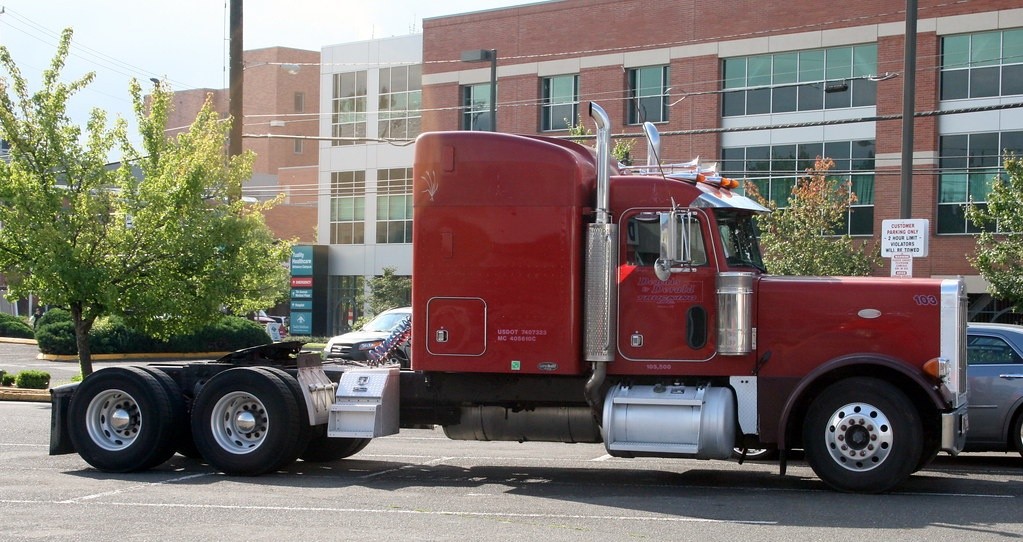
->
[271,316,289,328]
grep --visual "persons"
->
[30,308,43,328]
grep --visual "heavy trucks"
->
[50,103,967,492]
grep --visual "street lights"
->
[459,49,498,131]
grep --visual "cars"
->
[252,310,276,325]
[968,323,1023,457]
[322,307,409,368]
